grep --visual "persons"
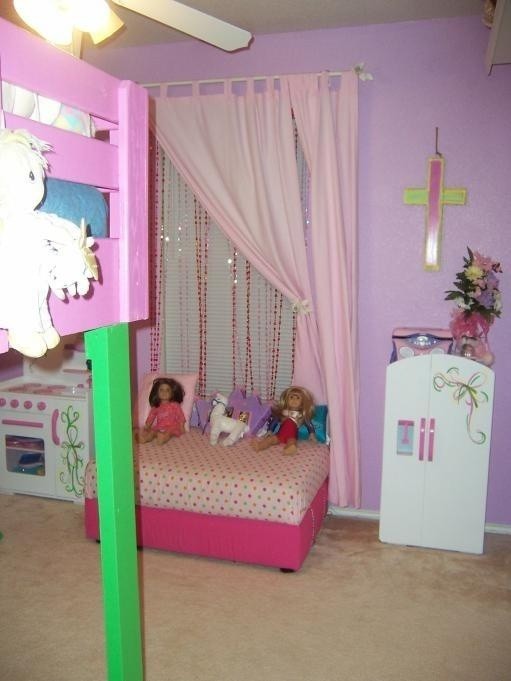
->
[254,385,318,455]
[134,377,186,446]
[0,125,98,359]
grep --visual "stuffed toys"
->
[239,410,249,424]
[207,393,250,447]
